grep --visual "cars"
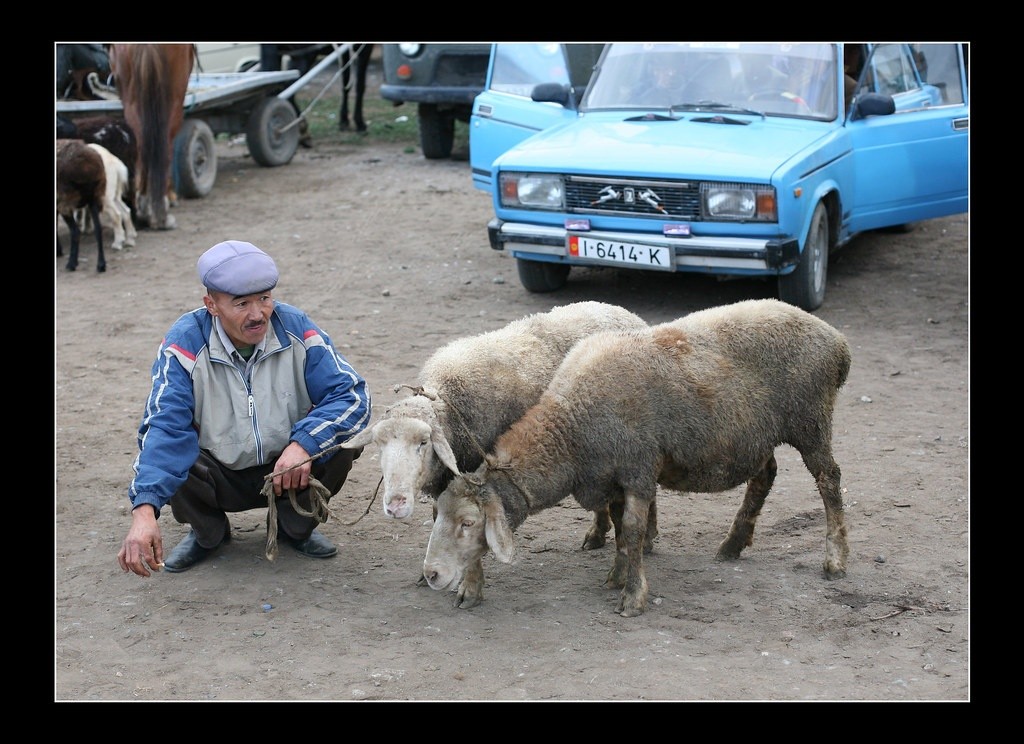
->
[467,42,971,312]
[377,41,489,160]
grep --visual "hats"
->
[197,240,278,295]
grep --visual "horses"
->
[110,43,195,231]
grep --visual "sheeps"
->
[54,119,138,274]
[341,300,659,587]
[423,299,858,618]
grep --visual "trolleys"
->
[58,42,362,200]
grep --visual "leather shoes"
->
[283,528,339,557]
[164,521,230,574]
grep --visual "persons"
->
[115,238,373,580]
[606,43,925,118]
